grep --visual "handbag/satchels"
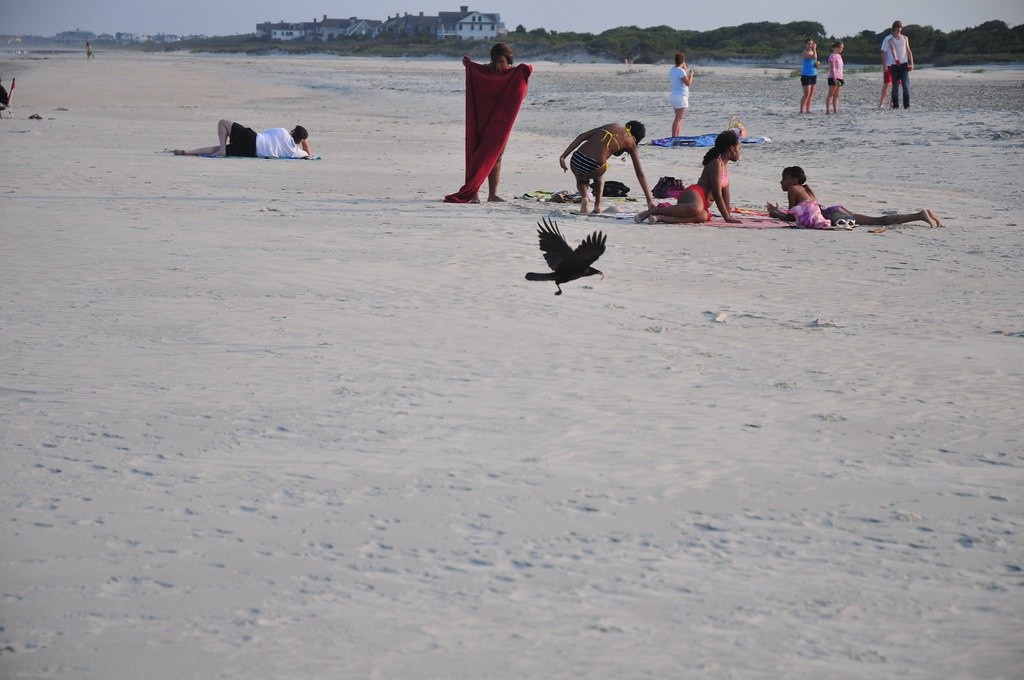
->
[590,181,630,197]
[651,177,685,199]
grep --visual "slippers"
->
[845,219,859,228]
[821,219,852,230]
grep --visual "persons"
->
[0,77,8,107]
[85,42,96,65]
[765,166,941,228]
[635,130,742,224]
[462,43,532,204]
[800,38,820,114]
[560,120,656,213]
[669,52,695,137]
[173,119,312,158]
[826,40,844,114]
[877,21,913,111]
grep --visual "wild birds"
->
[525,215,608,297]
